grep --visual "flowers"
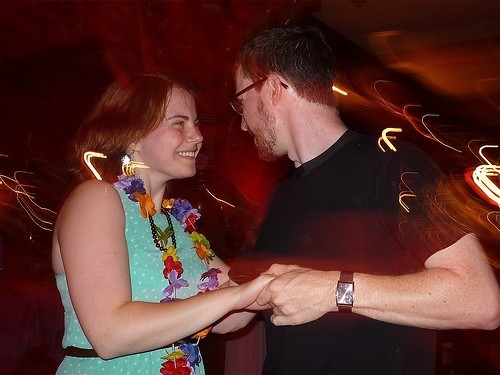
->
[111,160,229,375]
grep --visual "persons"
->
[49,69,278,374]
[203,21,499,374]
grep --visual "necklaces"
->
[146,209,178,253]
[112,172,222,375]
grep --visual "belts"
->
[63,334,207,361]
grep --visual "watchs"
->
[335,269,355,314]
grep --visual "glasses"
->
[228,76,289,117]
